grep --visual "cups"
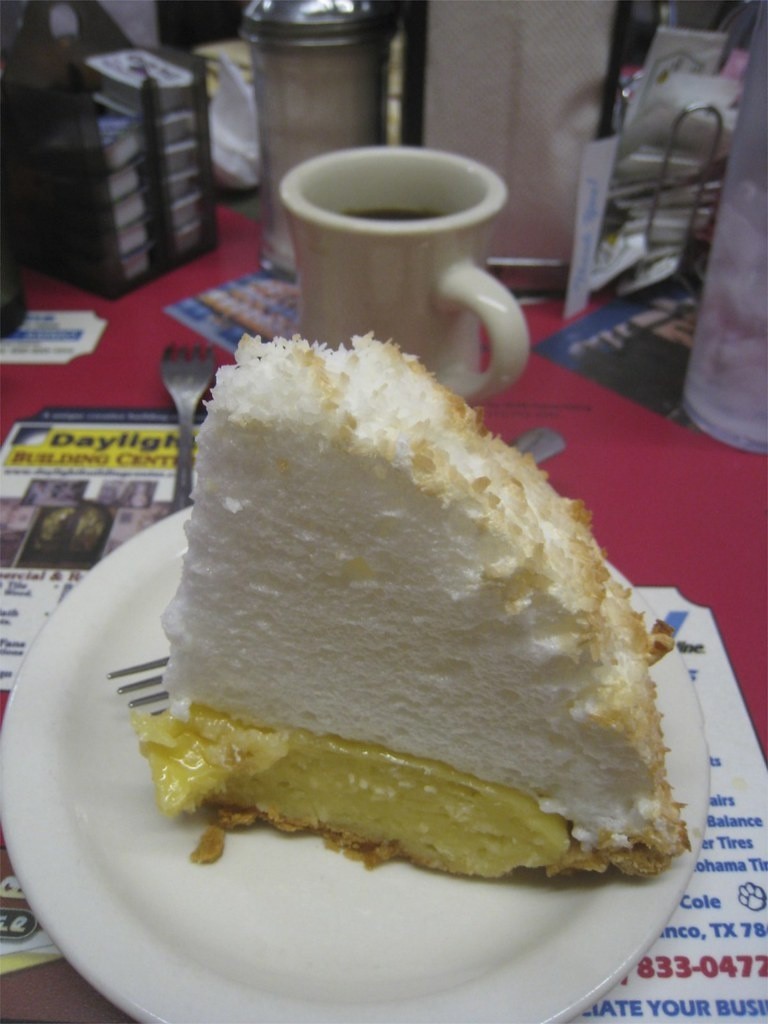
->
[279,147,530,404]
[681,0,768,456]
[237,0,389,282]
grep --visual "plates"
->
[0,505,712,1024]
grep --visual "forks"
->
[159,335,218,514]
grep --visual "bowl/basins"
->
[56,48,204,281]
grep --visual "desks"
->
[0,205,768,1024]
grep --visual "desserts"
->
[129,324,688,883]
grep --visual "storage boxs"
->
[1,1,217,300]
